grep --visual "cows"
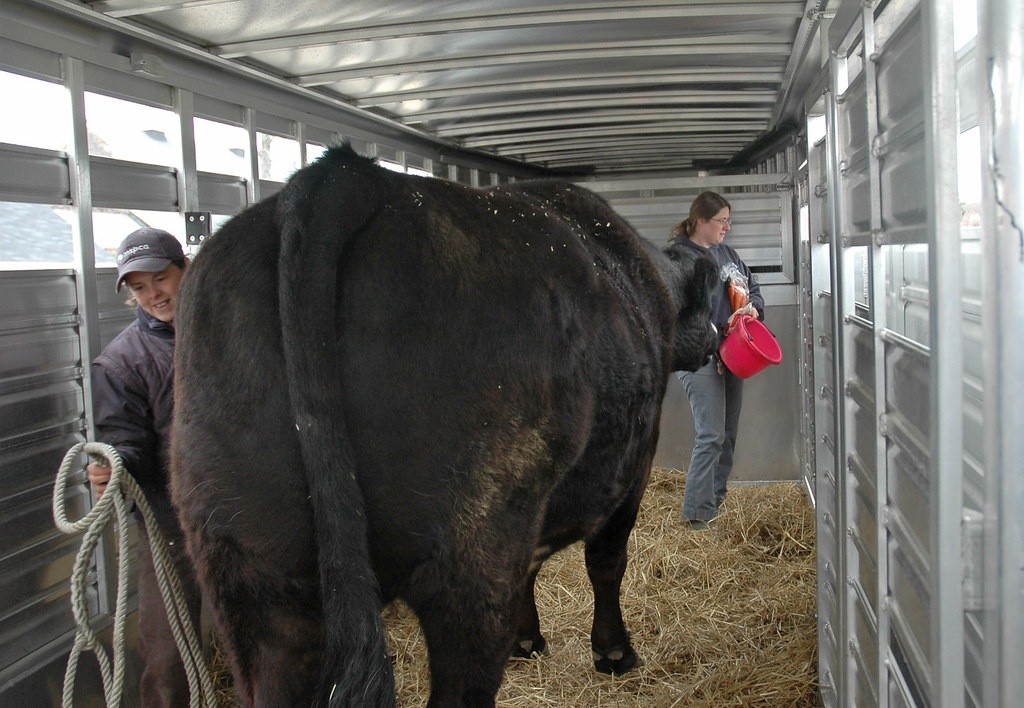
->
[166,142,721,708]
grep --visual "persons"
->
[667,191,764,531]
[87,227,203,708]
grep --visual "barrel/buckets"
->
[719,315,782,379]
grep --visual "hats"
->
[115,227,184,293]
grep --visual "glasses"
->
[703,215,733,226]
